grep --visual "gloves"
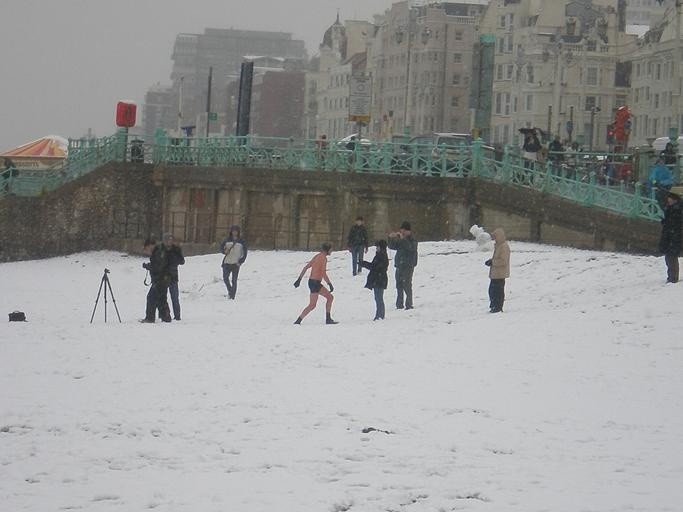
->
[328,283,334,293]
[485,260,492,266]
[294,277,301,288]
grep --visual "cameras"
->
[143,263,151,270]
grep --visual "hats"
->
[144,240,155,246]
[400,221,410,231]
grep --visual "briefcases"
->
[8,311,28,322]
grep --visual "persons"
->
[347,217,368,276]
[649,142,676,190]
[388,221,417,310]
[221,226,247,299]
[139,235,185,323]
[431,147,440,176]
[346,136,355,172]
[293,242,339,324]
[494,131,634,193]
[357,240,389,321]
[658,194,683,283]
[485,228,510,313]
[0,158,19,193]
[315,133,326,169]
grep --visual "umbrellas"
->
[518,126,536,133]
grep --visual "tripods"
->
[90,268,121,323]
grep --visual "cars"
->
[337,132,473,176]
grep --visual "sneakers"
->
[490,303,503,313]
[326,319,338,324]
